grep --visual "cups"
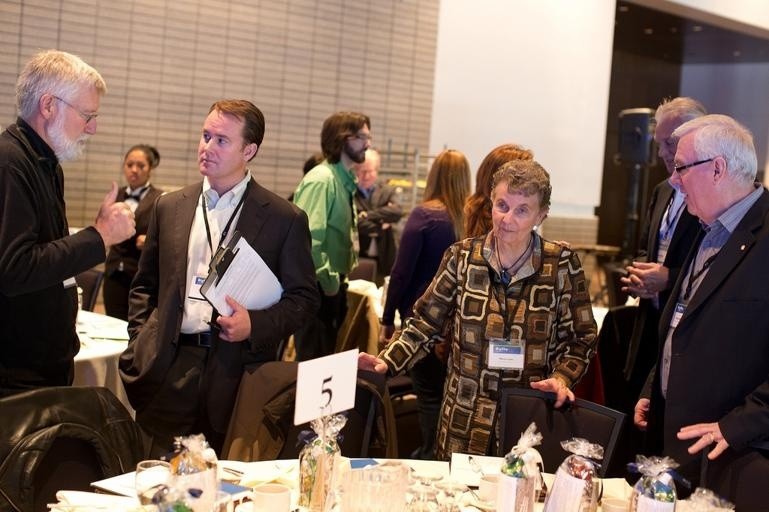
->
[479,474,500,502]
[381,276,390,306]
[601,498,630,512]
[498,463,534,511]
[254,484,290,511]
[134,461,174,490]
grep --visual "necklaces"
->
[494,233,533,287]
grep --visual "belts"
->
[177,331,214,346]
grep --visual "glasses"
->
[50,95,98,124]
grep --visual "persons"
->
[619,96,707,464]
[462,142,534,238]
[377,149,471,460]
[118,98,317,462]
[287,149,326,204]
[0,49,140,399]
[357,158,599,460]
[354,148,406,287]
[632,112,769,501]
[292,110,374,363]
[102,143,169,321]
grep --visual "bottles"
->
[296,448,338,512]
[543,453,599,512]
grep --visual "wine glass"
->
[336,466,467,512]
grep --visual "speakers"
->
[620,108,656,166]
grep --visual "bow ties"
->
[124,191,140,201]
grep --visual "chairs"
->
[335,291,422,416]
[0,385,143,511]
[501,388,627,477]
[220,358,400,460]
[597,307,663,398]
[74,269,106,312]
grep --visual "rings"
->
[708,432,715,442]
